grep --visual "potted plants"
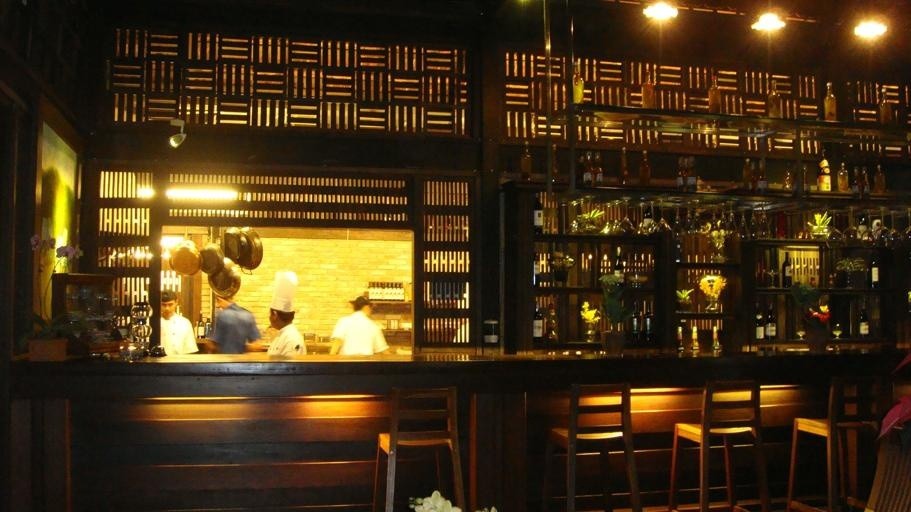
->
[806,210,833,241]
[595,273,634,350]
[674,286,694,310]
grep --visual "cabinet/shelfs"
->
[52,271,123,354]
[501,177,911,352]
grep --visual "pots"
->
[167,226,263,302]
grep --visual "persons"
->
[199,291,263,354]
[160,290,200,354]
[265,298,307,357]
[330,296,390,355]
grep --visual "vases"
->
[806,326,828,352]
[27,333,75,362]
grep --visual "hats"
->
[270,270,299,313]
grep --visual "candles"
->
[692,325,698,340]
[712,325,718,339]
[677,326,683,340]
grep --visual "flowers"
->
[25,234,84,338]
[802,303,832,327]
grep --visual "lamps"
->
[162,118,191,149]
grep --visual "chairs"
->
[665,378,769,512]
[369,385,472,512]
[540,383,641,512]
[788,379,888,511]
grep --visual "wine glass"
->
[794,324,806,340]
[830,322,843,338]
[670,205,771,240]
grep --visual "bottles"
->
[858,297,871,338]
[641,71,655,109]
[197,311,213,340]
[515,139,886,194]
[824,82,836,121]
[753,302,777,341]
[530,197,653,233]
[366,281,405,303]
[767,78,780,118]
[879,88,891,123]
[856,206,882,242]
[780,248,880,289]
[532,246,624,288]
[572,63,585,104]
[532,297,654,345]
[482,319,499,344]
[708,75,721,113]
